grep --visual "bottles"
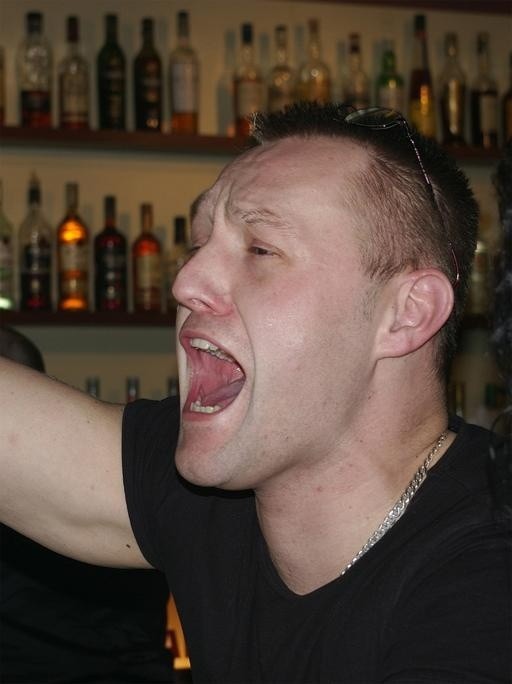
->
[442,379,467,424]
[1,165,195,321]
[83,376,178,404]
[469,201,511,318]
[1,7,511,146]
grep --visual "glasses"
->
[246,98,461,287]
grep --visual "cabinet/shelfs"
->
[0,124,512,326]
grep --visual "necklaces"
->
[340,430,453,580]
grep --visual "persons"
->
[0,103,512,684]
